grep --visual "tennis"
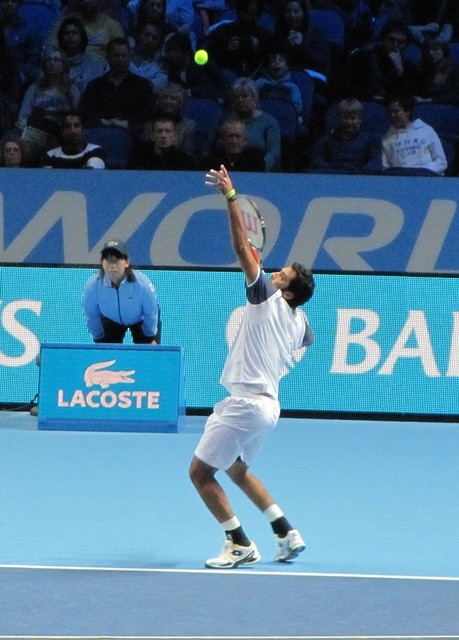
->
[194,49,209,65]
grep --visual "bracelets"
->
[224,188,237,201]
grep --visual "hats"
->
[100,239,128,255]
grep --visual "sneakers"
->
[205,540,261,569]
[273,529,306,562]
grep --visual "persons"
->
[311,95,382,174]
[414,36,459,106]
[82,239,162,345]
[351,20,420,101]
[185,164,316,570]
[1,1,334,172]
[379,79,449,176]
